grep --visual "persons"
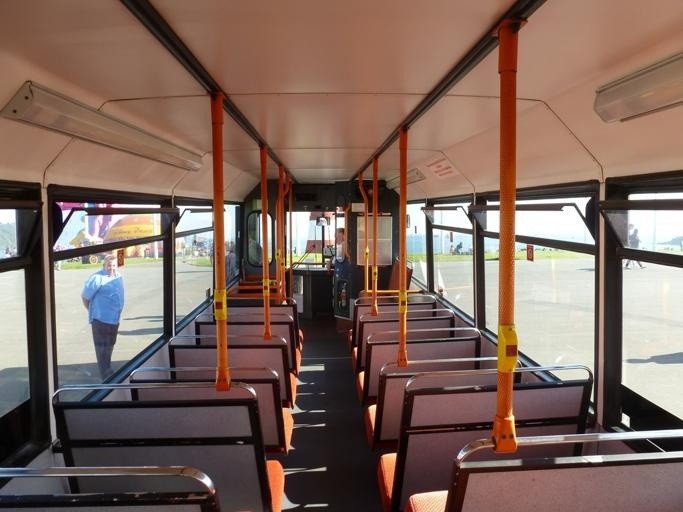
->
[207,241,238,284]
[248,236,264,265]
[326,228,347,296]
[3,245,80,272]
[80,255,124,380]
[449,242,453,252]
[456,242,463,254]
[624,228,647,269]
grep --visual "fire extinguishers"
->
[341,282,347,308]
